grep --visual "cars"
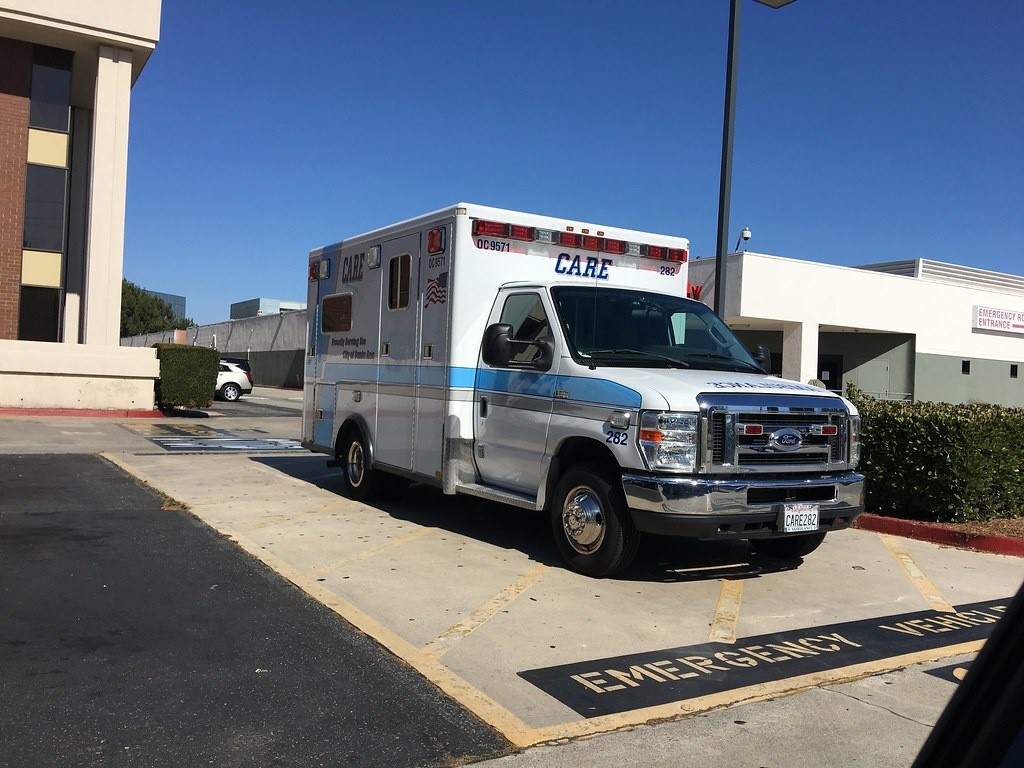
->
[214,360,255,402]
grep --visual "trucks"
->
[301,202,869,581]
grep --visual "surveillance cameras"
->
[742,230,751,241]
